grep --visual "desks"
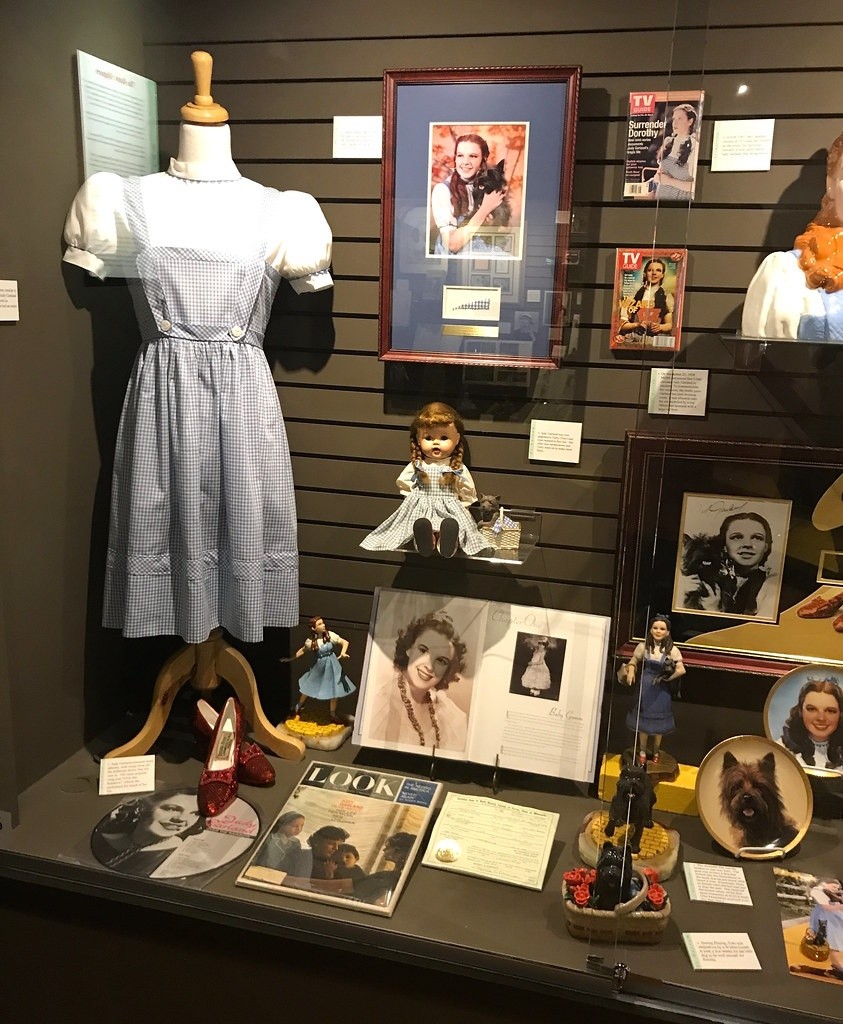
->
[0,707,843,1023]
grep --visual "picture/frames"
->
[607,430,843,684]
[611,247,691,354]
[670,492,793,625]
[816,550,843,585]
[373,60,587,373]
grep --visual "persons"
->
[778,675,843,768]
[252,810,417,907]
[628,613,686,764]
[431,134,514,257]
[93,787,208,878]
[654,104,698,199]
[809,879,843,971]
[359,403,495,559]
[683,512,778,618]
[521,636,551,696]
[369,610,468,752]
[63,100,334,643]
[280,616,357,722]
[620,259,674,337]
[741,133,843,343]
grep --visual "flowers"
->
[559,864,667,912]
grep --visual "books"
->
[235,760,443,919]
[609,248,688,353]
[622,91,704,200]
[350,586,611,786]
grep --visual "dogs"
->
[806,931,815,941]
[718,750,799,856]
[587,840,635,911]
[605,759,658,854]
[813,919,828,946]
[477,493,501,529]
[449,157,510,253]
[679,529,746,616]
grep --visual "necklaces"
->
[398,674,441,749]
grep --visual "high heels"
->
[192,699,278,788]
[197,697,250,816]
